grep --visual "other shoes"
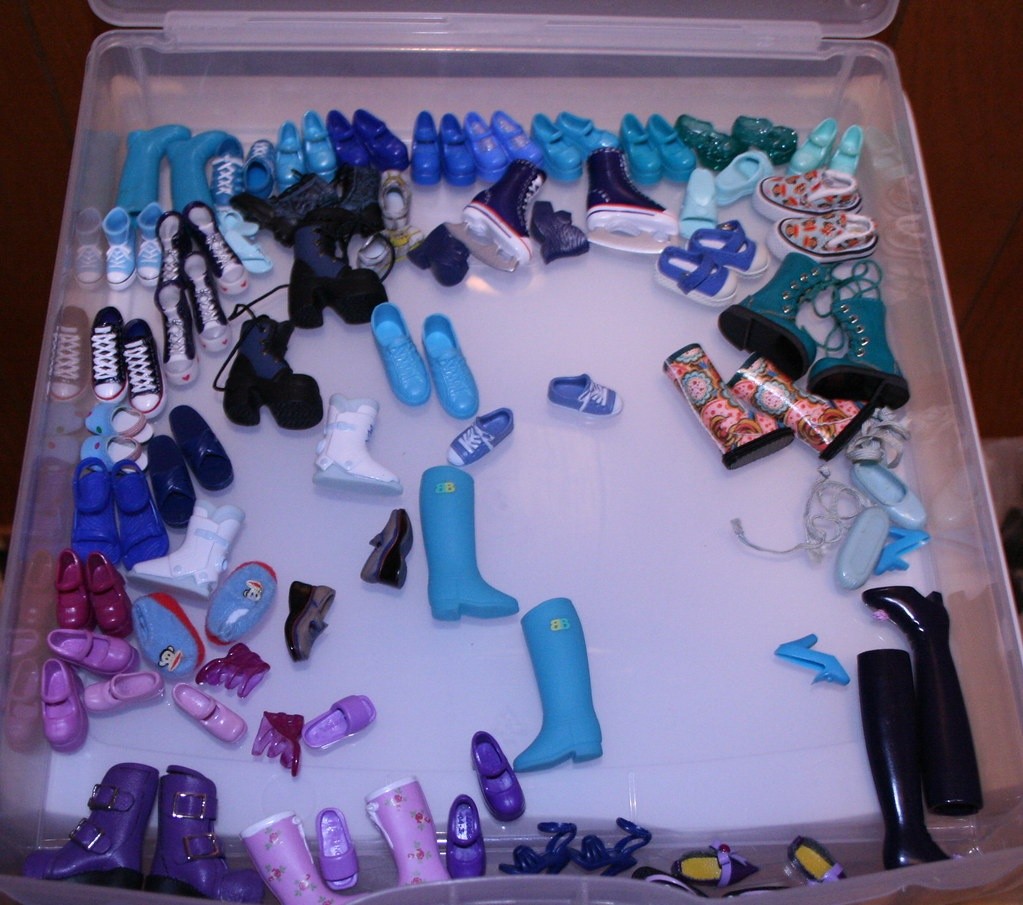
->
[25,105,985,905]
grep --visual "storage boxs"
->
[0,0,1023,905]
